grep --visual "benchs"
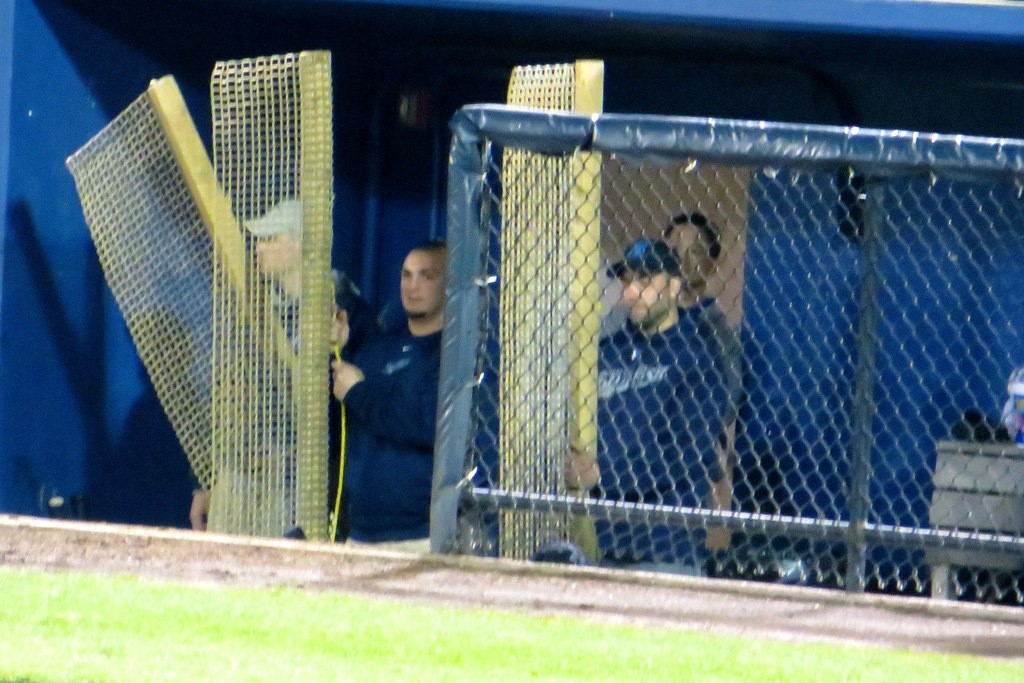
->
[929,437,1024,604]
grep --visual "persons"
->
[189,200,383,534]
[563,238,735,580]
[663,212,741,376]
[330,241,481,552]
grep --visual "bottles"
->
[778,549,808,586]
[1007,359,1024,448]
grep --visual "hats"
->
[244,199,304,237]
[607,238,679,276]
